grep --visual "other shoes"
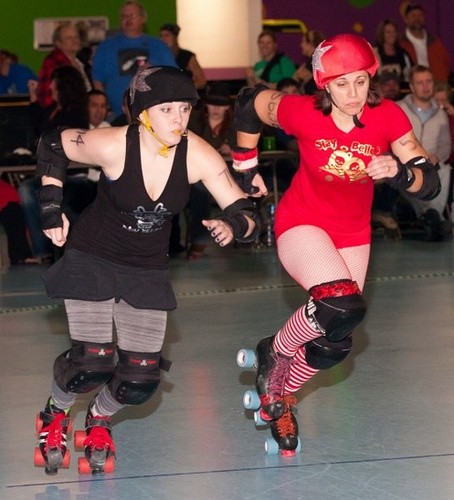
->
[0,209,454,264]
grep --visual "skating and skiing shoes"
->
[237,335,292,418]
[35,396,72,473]
[74,393,115,476]
[255,410,302,456]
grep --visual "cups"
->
[263,137,275,151]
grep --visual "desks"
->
[0,150,299,259]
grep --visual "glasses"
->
[121,14,141,18]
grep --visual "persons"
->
[32,67,263,474]
[1,3,454,269]
[232,32,443,458]
[392,65,454,240]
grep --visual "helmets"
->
[128,66,200,119]
[313,33,378,90]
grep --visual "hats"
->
[203,86,232,106]
[88,29,105,42]
[161,24,180,34]
[406,4,423,12]
[379,73,400,84]
